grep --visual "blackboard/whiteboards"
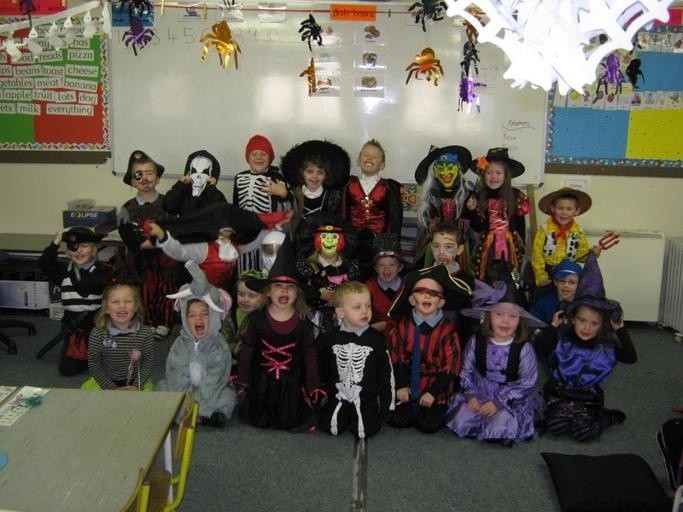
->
[110,0,547,188]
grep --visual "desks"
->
[0,380,187,509]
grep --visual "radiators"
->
[658,235,682,342]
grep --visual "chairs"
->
[157,401,201,511]
[110,462,171,511]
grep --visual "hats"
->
[241,269,266,281]
[459,259,550,328]
[245,230,321,299]
[538,187,591,216]
[371,232,402,264]
[123,150,164,185]
[63,227,108,243]
[246,135,274,164]
[415,145,471,185]
[104,265,143,285]
[470,148,525,178]
[387,263,473,317]
[551,249,621,322]
[282,141,350,190]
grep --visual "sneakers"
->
[202,412,225,427]
[598,407,626,425]
[150,326,170,340]
